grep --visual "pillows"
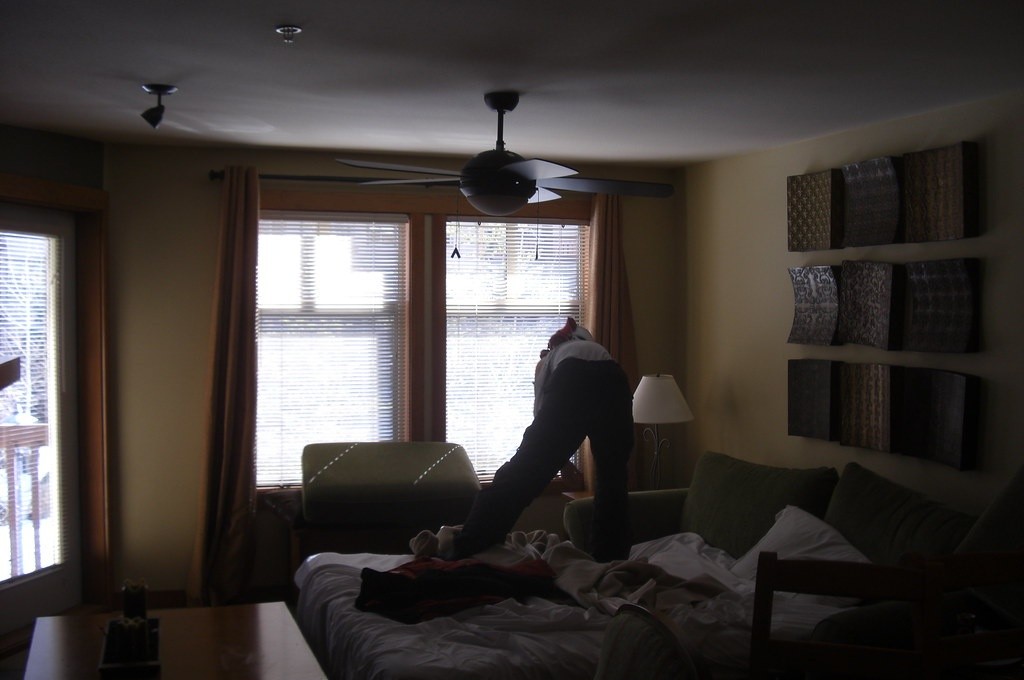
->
[301,440,483,528]
[681,449,839,560]
[823,460,979,569]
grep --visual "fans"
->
[336,90,675,218]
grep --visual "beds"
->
[293,447,980,680]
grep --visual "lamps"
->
[630,374,695,492]
[140,83,178,129]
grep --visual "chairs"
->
[590,602,713,680]
[921,542,1024,680]
[745,547,938,679]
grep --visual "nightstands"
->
[558,489,594,508]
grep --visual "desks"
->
[23,601,326,680]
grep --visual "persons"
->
[453,339,632,563]
